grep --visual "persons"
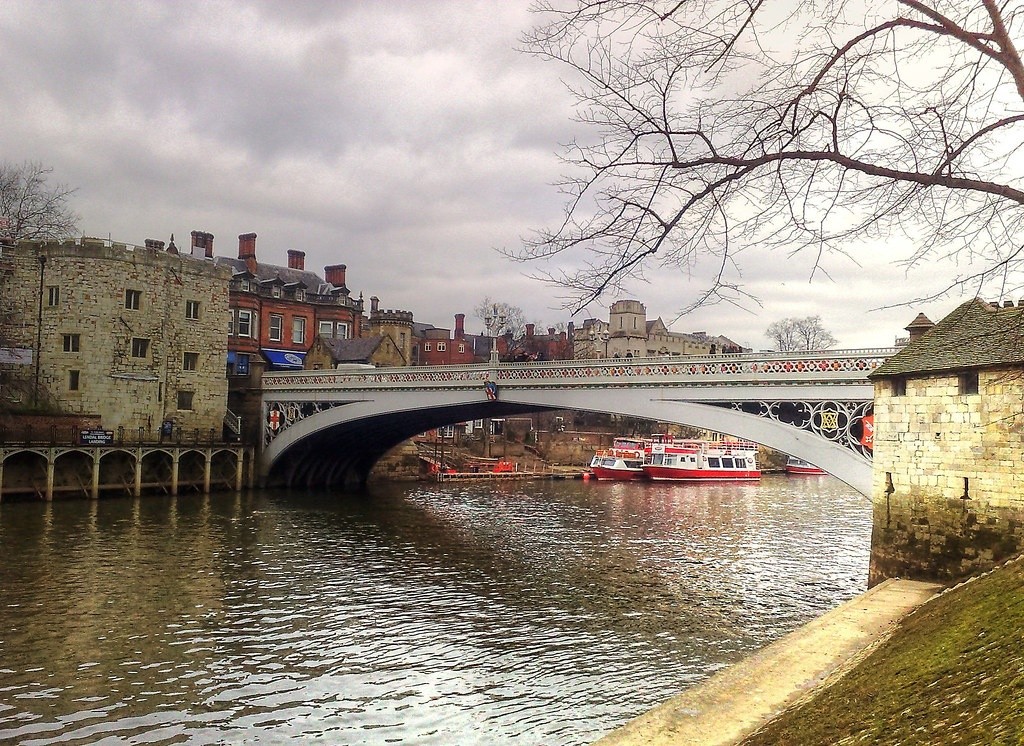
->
[501,351,548,362]
[612,349,633,376]
[707,345,742,372]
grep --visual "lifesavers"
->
[634,452,640,459]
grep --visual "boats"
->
[640,433,762,481]
[784,455,828,475]
[589,439,722,480]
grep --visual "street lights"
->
[482,304,504,361]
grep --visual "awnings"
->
[261,348,306,369]
